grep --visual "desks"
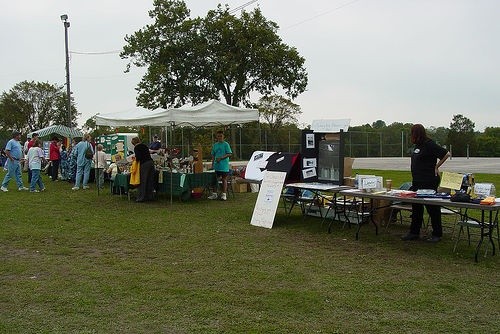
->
[115,174,136,200]
[285,182,356,234]
[340,189,500,262]
[154,168,221,200]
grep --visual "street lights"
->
[60,15,72,129]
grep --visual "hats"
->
[153,133,160,138]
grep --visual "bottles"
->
[355,172,359,187]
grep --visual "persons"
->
[131,137,154,202]
[43,135,94,190]
[0,132,45,192]
[107,154,122,193]
[208,131,232,200]
[403,124,450,241]
[93,144,107,188]
[149,136,165,153]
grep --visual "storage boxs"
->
[230,183,247,193]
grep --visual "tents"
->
[96,100,261,204]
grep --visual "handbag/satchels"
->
[450,192,472,203]
[85,147,94,159]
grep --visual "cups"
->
[187,168,191,173]
[184,169,187,173]
[386,180,392,191]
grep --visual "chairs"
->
[385,181,423,236]
[423,172,474,240]
[451,198,500,258]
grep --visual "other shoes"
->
[72,186,80,190]
[208,193,218,200]
[427,236,442,242]
[1,186,9,191]
[30,189,40,193]
[401,231,419,240]
[19,186,29,191]
[82,185,90,190]
[221,192,227,201]
[41,186,46,192]
[136,198,145,202]
